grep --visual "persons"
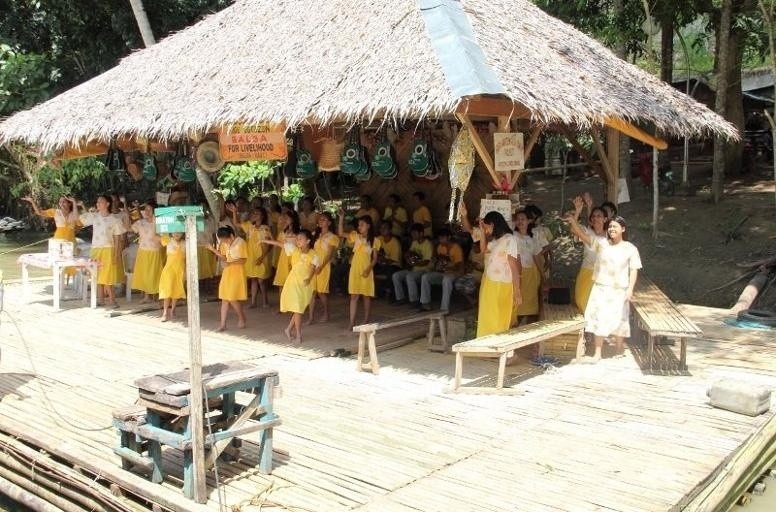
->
[459,195,553,358]
[25,193,215,322]
[203,194,484,344]
[556,194,644,363]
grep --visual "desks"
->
[16,253,100,311]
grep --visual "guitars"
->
[371,123,400,180]
[284,129,319,180]
[142,142,174,182]
[172,141,198,182]
[339,125,371,182]
[105,135,125,171]
[407,121,443,180]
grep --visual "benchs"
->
[352,309,449,375]
[451,319,588,390]
[629,274,704,374]
[539,277,584,358]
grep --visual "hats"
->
[196,141,224,172]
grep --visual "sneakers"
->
[418,303,433,311]
[392,299,406,306]
[409,300,419,308]
